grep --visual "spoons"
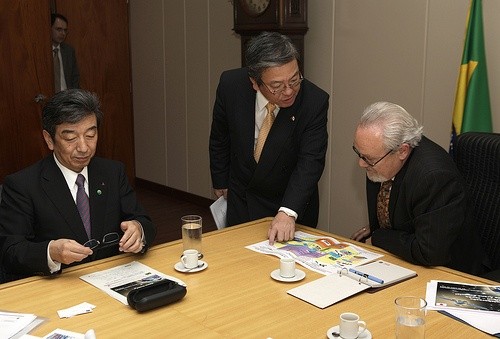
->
[184,262,204,272]
[332,331,341,337]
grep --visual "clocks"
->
[232,0,309,78]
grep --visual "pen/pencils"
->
[349,268,384,284]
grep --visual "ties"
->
[255,102,276,165]
[75,174,90,240]
[53,48,63,94]
[377,180,393,231]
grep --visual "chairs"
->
[452,131,500,281]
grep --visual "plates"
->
[271,269,306,282]
[174,260,208,273]
[327,326,372,339]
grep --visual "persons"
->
[0,90,156,284]
[351,102,492,276]
[208,32,330,245]
[51,13,81,94]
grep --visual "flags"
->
[449,0,493,157]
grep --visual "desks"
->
[0,215,500,339]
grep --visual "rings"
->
[363,228,367,231]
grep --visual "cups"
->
[181,215,203,260]
[181,249,198,269]
[280,258,295,278]
[339,312,366,339]
[393,296,427,339]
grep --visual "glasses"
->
[352,146,393,167]
[258,72,304,95]
[79,232,124,251]
